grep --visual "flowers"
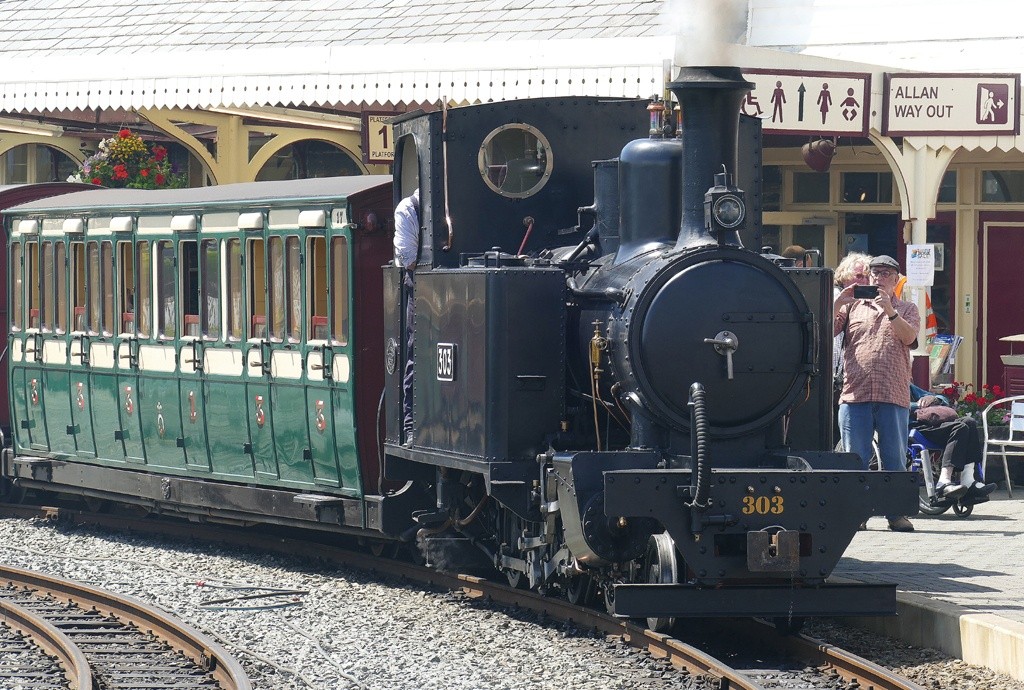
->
[66,129,190,189]
[940,381,1012,426]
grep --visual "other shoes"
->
[959,480,997,504]
[407,433,413,447]
[936,482,968,498]
[886,516,915,532]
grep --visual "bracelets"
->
[887,312,898,320]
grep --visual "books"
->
[928,333,963,373]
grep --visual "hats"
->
[868,255,901,276]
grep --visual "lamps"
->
[801,135,837,172]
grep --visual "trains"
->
[0,64,924,633]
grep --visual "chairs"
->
[981,395,1024,497]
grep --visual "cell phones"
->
[854,285,879,299]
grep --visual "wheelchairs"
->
[835,399,975,519]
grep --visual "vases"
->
[976,427,1024,486]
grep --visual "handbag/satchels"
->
[913,404,960,425]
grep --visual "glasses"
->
[868,270,897,279]
[853,273,869,280]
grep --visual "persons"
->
[907,384,998,497]
[393,187,420,447]
[781,245,921,533]
